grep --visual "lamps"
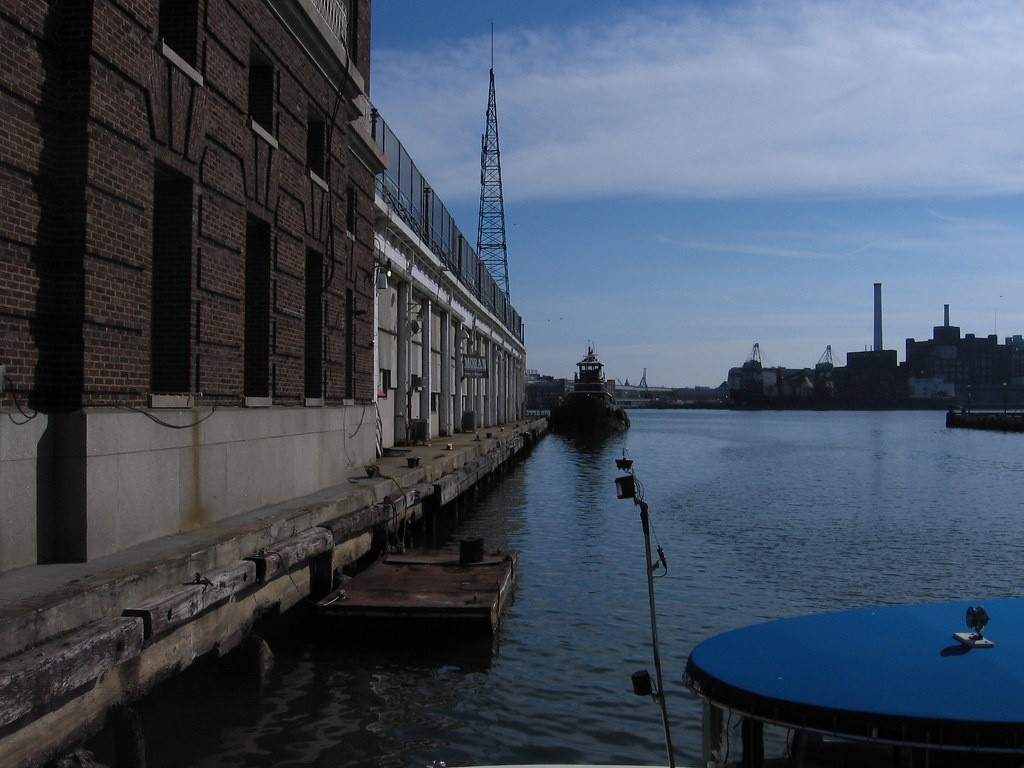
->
[408,311,425,322]
[369,261,390,290]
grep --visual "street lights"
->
[1002,376,1007,415]
[967,383,971,414]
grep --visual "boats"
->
[615,447,1024,768]
[545,340,631,444]
